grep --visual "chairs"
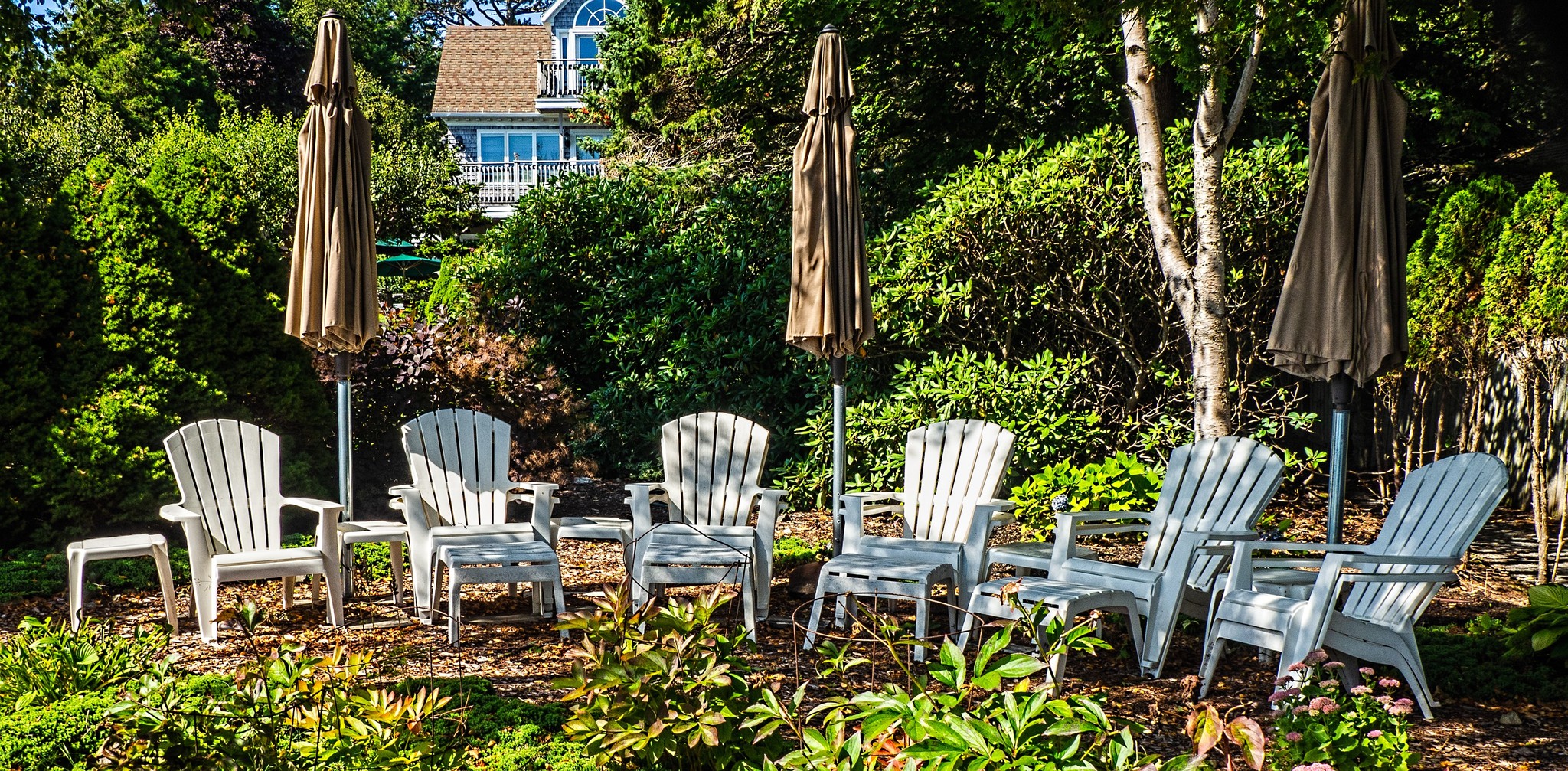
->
[159,415,349,646]
[837,417,1017,640]
[1042,432,1288,682]
[621,409,788,626]
[1188,451,1511,725]
[388,406,559,626]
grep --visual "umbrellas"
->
[280,16,382,607]
[1260,1,1413,553]
[778,23,878,562]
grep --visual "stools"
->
[955,570,1150,700]
[634,540,758,652]
[977,546,1108,650]
[547,515,636,607]
[311,516,420,619]
[430,540,574,648]
[1208,559,1348,674]
[805,553,960,664]
[66,533,181,640]
